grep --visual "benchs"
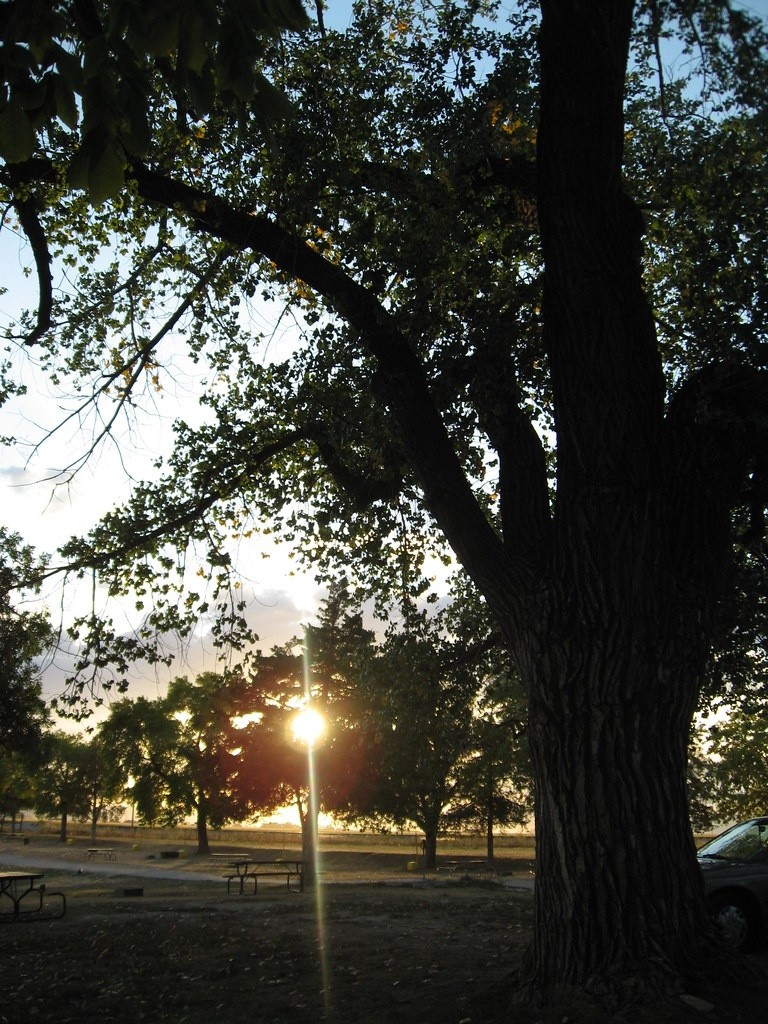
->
[436,860,497,880]
[84,848,117,861]
[222,859,310,896]
[0,870,68,917]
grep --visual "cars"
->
[694,816,767,951]
